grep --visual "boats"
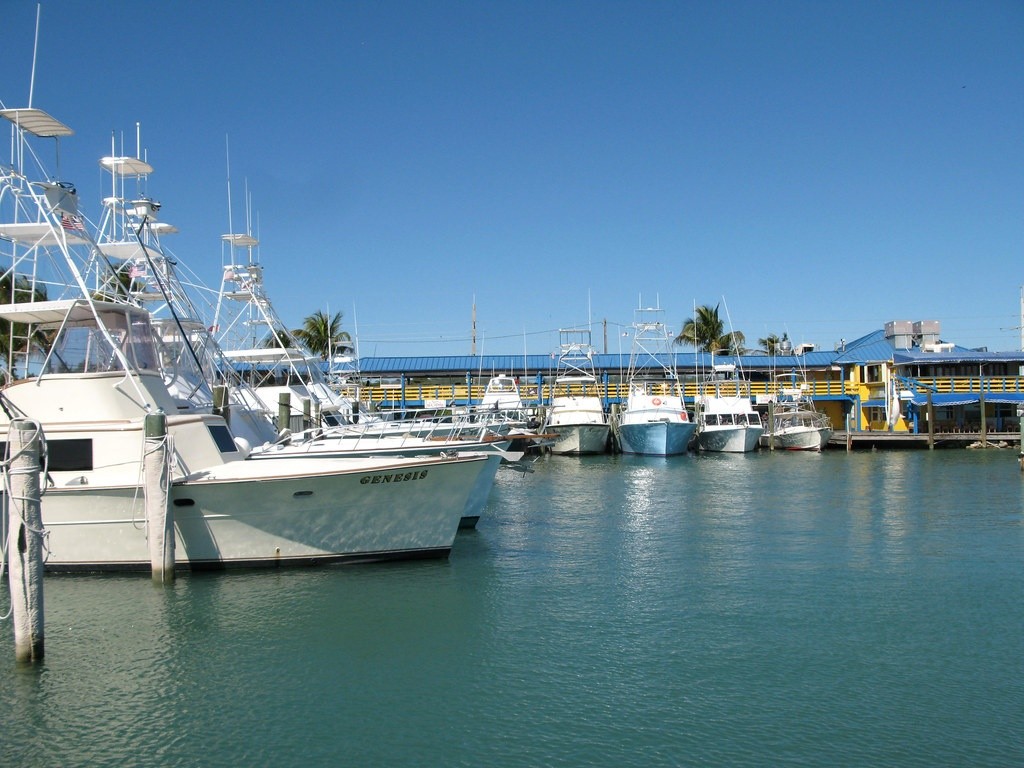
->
[614,290,700,457]
[43,121,540,531]
[542,289,613,456]
[0,4,490,571]
[758,323,834,452]
[692,294,763,453]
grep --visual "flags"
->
[224,271,234,282]
[60,215,84,230]
[130,264,148,278]
